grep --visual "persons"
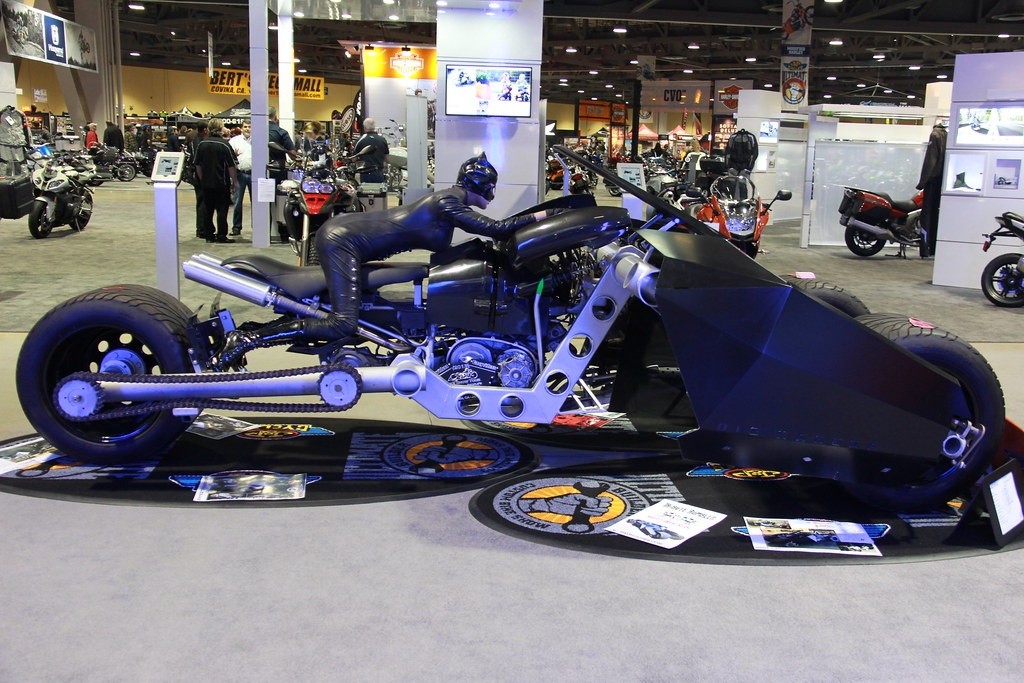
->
[33,126,65,144]
[708,131,712,149]
[501,72,527,95]
[210,152,576,373]
[589,139,606,153]
[653,143,673,157]
[193,106,390,244]
[414,90,436,104]
[84,118,334,158]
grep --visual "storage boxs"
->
[54,135,81,151]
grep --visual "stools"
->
[0,174,34,220]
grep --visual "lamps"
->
[613,23,628,33]
[221,60,231,66]
[365,44,374,50]
[829,36,843,45]
[128,2,146,10]
[401,45,411,51]
[566,45,578,53]
[295,56,300,62]
[873,53,886,58]
[687,40,700,49]
[630,59,639,64]
[936,70,948,79]
[268,22,278,30]
[353,45,359,51]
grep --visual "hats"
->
[456,151,498,201]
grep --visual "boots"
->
[206,320,304,372]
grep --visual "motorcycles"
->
[980,211,1024,307]
[545,144,793,260]
[271,127,435,267]
[24,130,136,238]
[838,186,924,259]
[15,147,1007,513]
[79,40,90,54]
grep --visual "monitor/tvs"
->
[445,64,532,118]
[151,152,184,182]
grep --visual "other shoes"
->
[206,234,217,242]
[216,236,235,243]
[196,230,207,238]
[229,229,240,235]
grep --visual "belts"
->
[237,170,251,174]
